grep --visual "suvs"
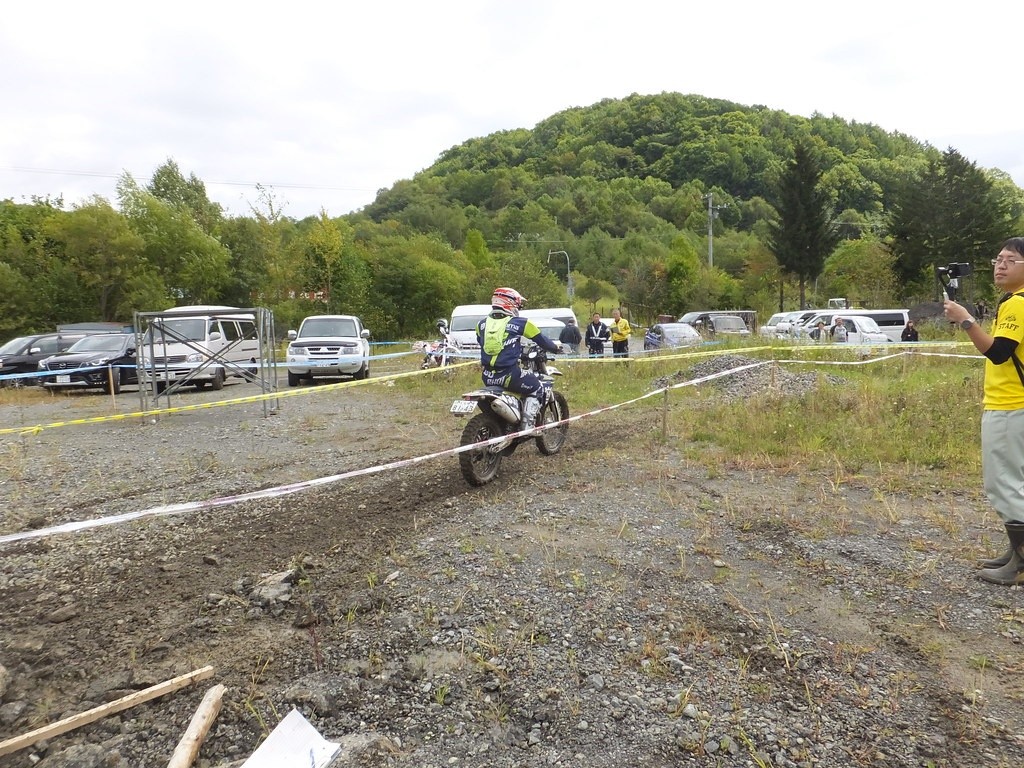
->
[286,314,371,386]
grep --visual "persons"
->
[830,317,849,342]
[943,236,1024,585]
[610,310,631,367]
[901,320,917,341]
[476,287,562,436]
[559,319,582,359]
[585,312,611,358]
[809,322,828,342]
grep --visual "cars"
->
[37,333,144,394]
[704,316,752,340]
[758,312,888,343]
[528,319,572,354]
[644,323,703,351]
[0,335,89,389]
[594,318,615,353]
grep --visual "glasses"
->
[990,257,1024,268]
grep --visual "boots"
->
[520,397,544,436]
[977,542,1013,568]
[981,522,1024,584]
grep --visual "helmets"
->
[436,319,448,329]
[491,287,528,318]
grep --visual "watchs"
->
[960,316,976,330]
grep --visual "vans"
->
[136,304,260,394]
[677,311,756,337]
[449,304,493,358]
[518,308,578,328]
[787,308,910,342]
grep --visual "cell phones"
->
[948,263,971,277]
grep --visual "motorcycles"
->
[418,327,460,369]
[455,344,572,486]
